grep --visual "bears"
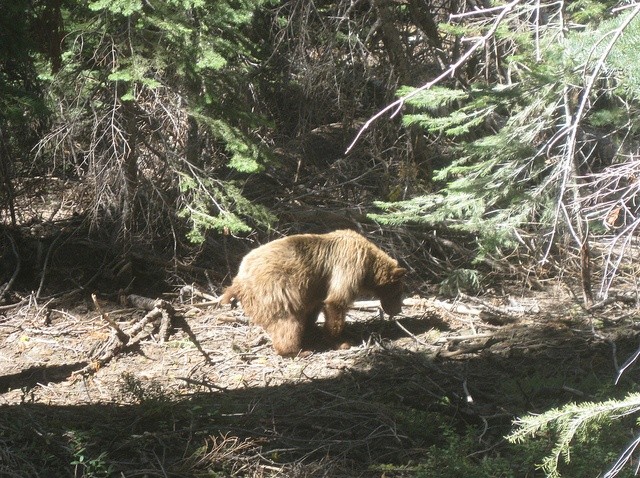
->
[220,225,416,360]
